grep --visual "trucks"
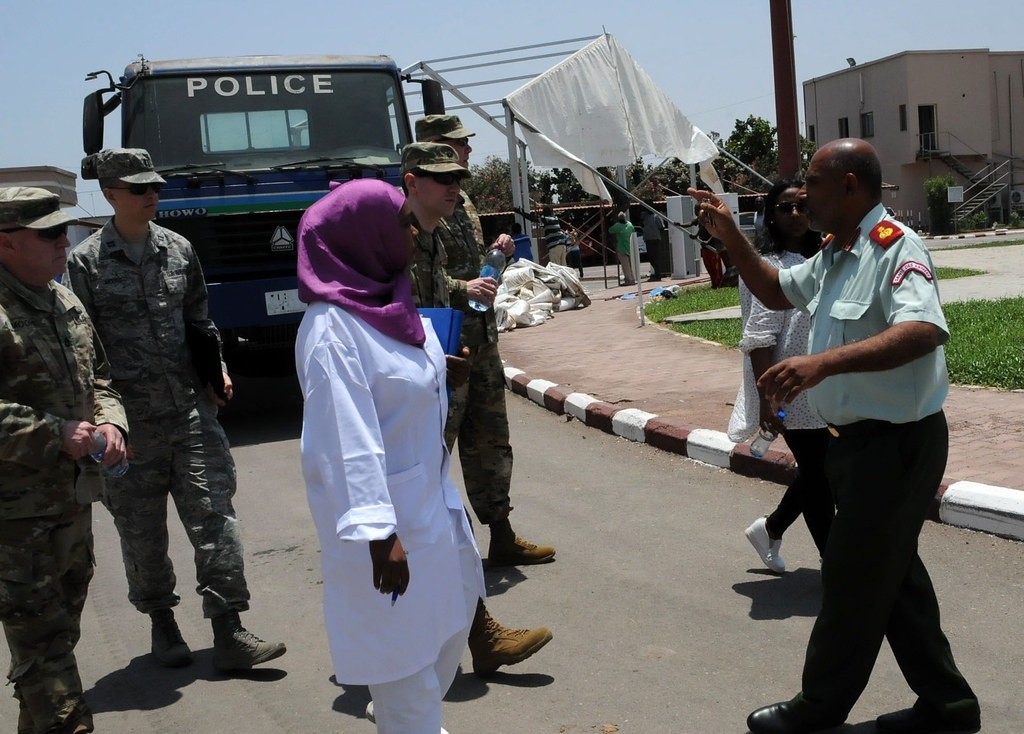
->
[77,53,448,415]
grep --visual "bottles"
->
[468,247,506,312]
[750,412,785,457]
[88,432,129,479]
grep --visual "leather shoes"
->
[876,706,982,734]
[747,701,814,734]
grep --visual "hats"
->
[415,114,476,145]
[0,187,79,230]
[617,211,626,220]
[400,142,472,180]
[97,147,168,184]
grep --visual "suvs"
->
[634,226,647,254]
[564,225,597,258]
[739,211,757,245]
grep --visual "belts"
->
[826,419,893,438]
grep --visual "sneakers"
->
[745,514,784,574]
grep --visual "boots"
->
[210,612,266,670]
[151,608,194,668]
[466,598,552,677]
[488,521,555,568]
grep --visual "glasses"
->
[0,223,68,240]
[412,168,462,186]
[775,201,808,215]
[436,137,469,146]
[103,182,163,195]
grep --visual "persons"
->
[563,226,584,278]
[728,177,836,573]
[608,211,635,286]
[415,113,556,564]
[642,198,665,281]
[0,187,130,734]
[59,149,289,668]
[688,138,983,734]
[295,177,487,734]
[513,205,567,266]
[511,223,533,263]
[674,203,733,269]
[399,142,554,677]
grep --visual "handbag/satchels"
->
[183,319,230,404]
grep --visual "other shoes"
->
[620,277,635,286]
[648,274,661,281]
